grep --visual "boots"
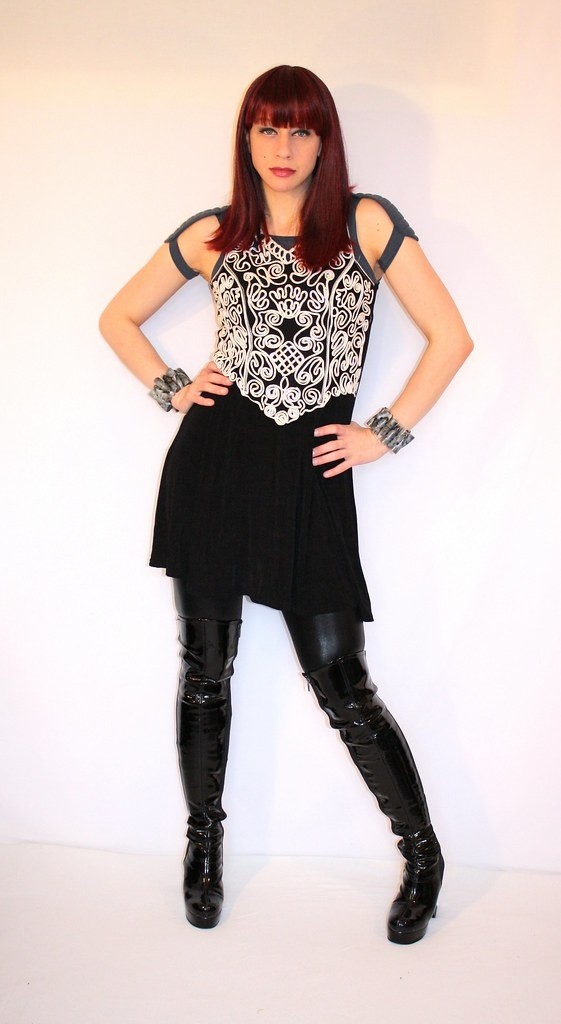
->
[176,616,243,929]
[301,648,444,946]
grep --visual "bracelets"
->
[367,407,415,454]
[152,367,191,412]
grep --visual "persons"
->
[100,64,473,945]
